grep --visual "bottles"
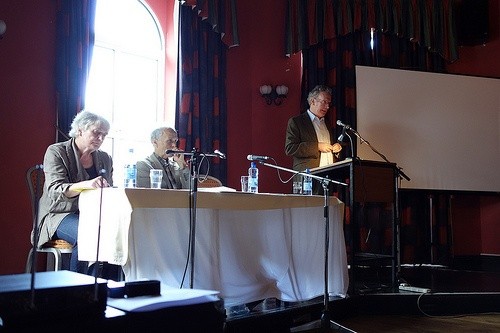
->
[248,162,258,193]
[303,169,312,195]
[125,149,137,188]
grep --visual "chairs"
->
[25,164,74,274]
[195,174,223,188]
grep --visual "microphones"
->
[336,120,353,130]
[247,155,270,161]
[213,149,227,159]
[342,130,355,159]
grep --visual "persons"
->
[285,86,344,198]
[31,112,118,281]
[136,128,193,190]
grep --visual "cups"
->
[241,176,251,192]
[150,170,163,188]
[293,176,302,194]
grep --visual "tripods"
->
[259,161,360,333]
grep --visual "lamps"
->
[259,84,288,105]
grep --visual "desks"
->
[78,188,348,309]
